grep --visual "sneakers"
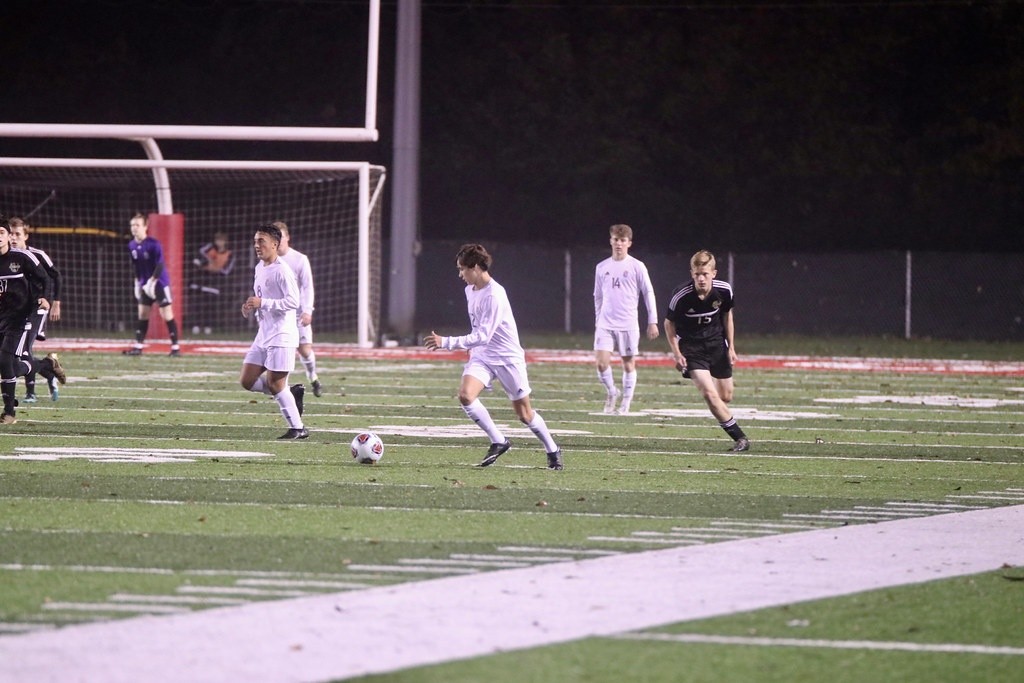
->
[546,447,563,471]
[481,437,512,466]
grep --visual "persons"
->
[422,243,564,469]
[0,217,67,425]
[664,249,749,451]
[591,225,659,412]
[273,222,322,396]
[238,224,310,441]
[121,212,182,357]
[189,230,237,335]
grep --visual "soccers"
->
[351,432,384,465]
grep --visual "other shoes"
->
[727,436,750,452]
[276,428,309,442]
[192,326,200,334]
[0,413,17,424]
[311,381,323,398]
[48,352,66,384]
[51,386,58,401]
[204,327,211,335]
[603,388,621,412]
[22,394,38,403]
[619,400,631,414]
[123,345,142,355]
[168,350,181,357]
[290,384,305,418]
